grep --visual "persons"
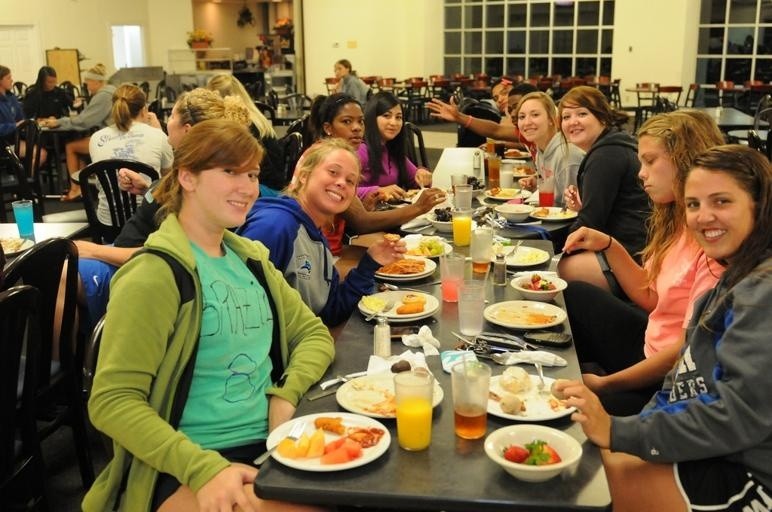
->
[557,85,659,304]
[562,108,732,416]
[0,48,448,256]
[51,89,253,361]
[517,88,592,206]
[423,81,541,165]
[81,118,336,511]
[556,145,772,510]
[491,76,520,126]
[235,138,406,329]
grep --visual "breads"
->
[513,166,534,176]
[504,148,521,157]
[403,294,426,304]
[397,303,423,314]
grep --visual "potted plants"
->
[186,29,214,49]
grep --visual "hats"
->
[85,63,109,81]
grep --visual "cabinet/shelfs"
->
[168,47,233,72]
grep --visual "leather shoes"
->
[60,187,84,203]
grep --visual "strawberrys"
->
[502,443,560,464]
[522,274,555,291]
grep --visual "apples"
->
[419,237,445,258]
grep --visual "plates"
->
[399,238,454,258]
[530,206,577,220]
[491,245,549,266]
[486,189,531,200]
[359,291,442,323]
[510,276,567,301]
[499,171,539,176]
[0,237,35,256]
[426,212,452,232]
[336,374,445,420]
[377,256,436,281]
[485,300,567,330]
[472,373,579,422]
[268,413,392,471]
[494,204,532,223]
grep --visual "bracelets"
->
[605,234,613,251]
[463,114,474,128]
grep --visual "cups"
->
[499,164,516,188]
[492,255,507,287]
[451,362,488,441]
[451,173,468,195]
[11,199,35,238]
[536,183,555,206]
[454,184,474,211]
[440,256,465,303]
[487,158,501,181]
[471,227,492,273]
[452,208,473,246]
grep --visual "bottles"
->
[372,318,391,358]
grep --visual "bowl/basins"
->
[484,424,583,483]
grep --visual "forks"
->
[267,420,307,456]
[550,249,567,271]
